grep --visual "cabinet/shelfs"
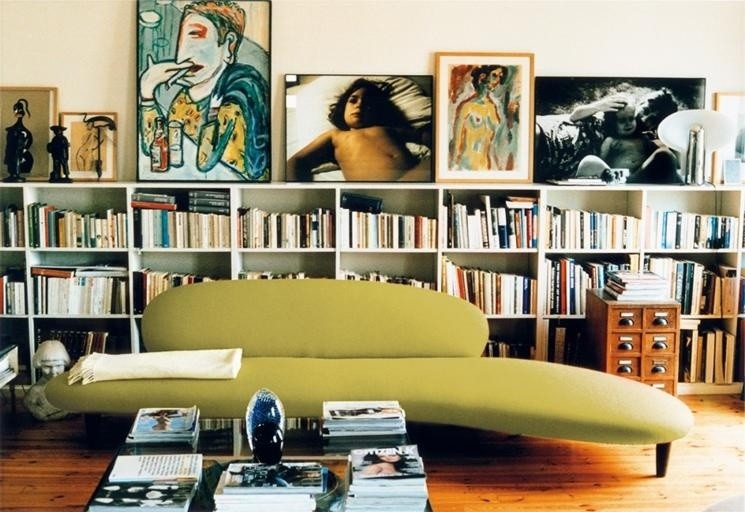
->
[586,286,680,395]
[2,181,744,395]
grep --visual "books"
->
[125,405,203,445]
[88,453,328,512]
[0,188,745,386]
[321,399,428,512]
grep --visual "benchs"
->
[44,280,694,478]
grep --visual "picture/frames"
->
[433,51,534,185]
[1,86,116,183]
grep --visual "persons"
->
[286,78,432,181]
[451,66,520,172]
[140,1,270,179]
[534,85,683,186]
[46,124,70,182]
[23,340,74,420]
